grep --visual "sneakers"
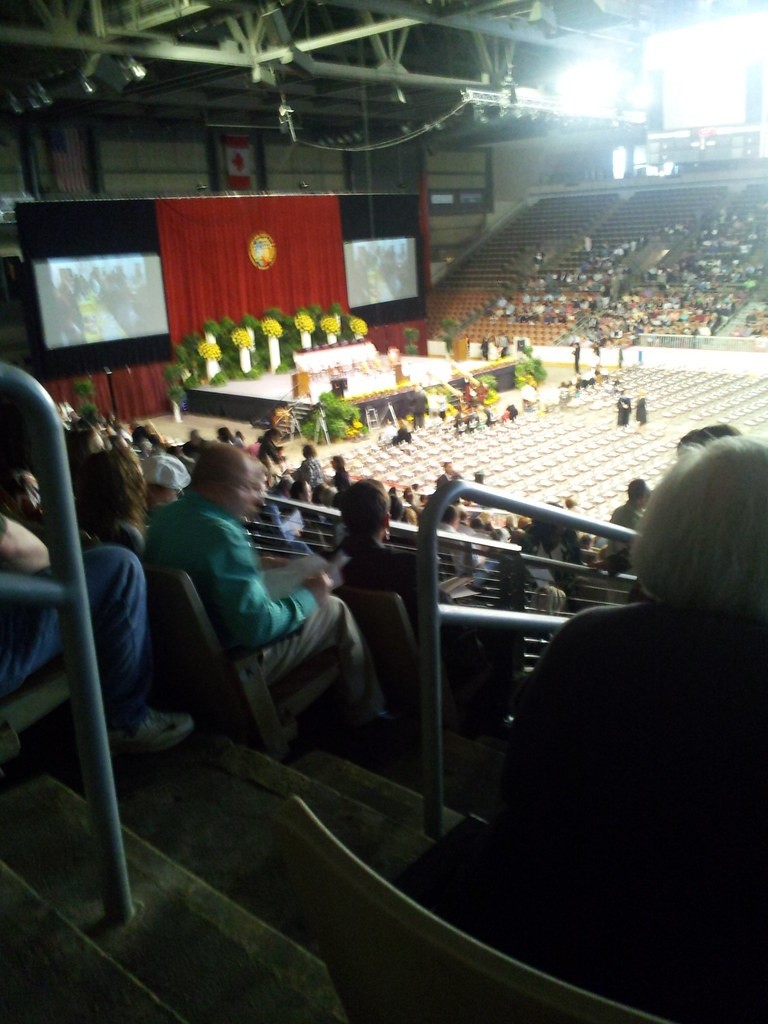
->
[104,704,197,757]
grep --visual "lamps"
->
[6,55,147,114]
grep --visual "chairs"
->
[273,791,675,1024]
[0,181,768,773]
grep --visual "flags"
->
[225,133,252,190]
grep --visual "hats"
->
[140,451,192,493]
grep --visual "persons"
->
[408,384,447,429]
[561,341,625,399]
[615,388,648,433]
[484,208,768,351]
[521,378,538,408]
[355,244,407,304]
[480,332,510,361]
[0,395,768,1024]
[54,262,147,344]
